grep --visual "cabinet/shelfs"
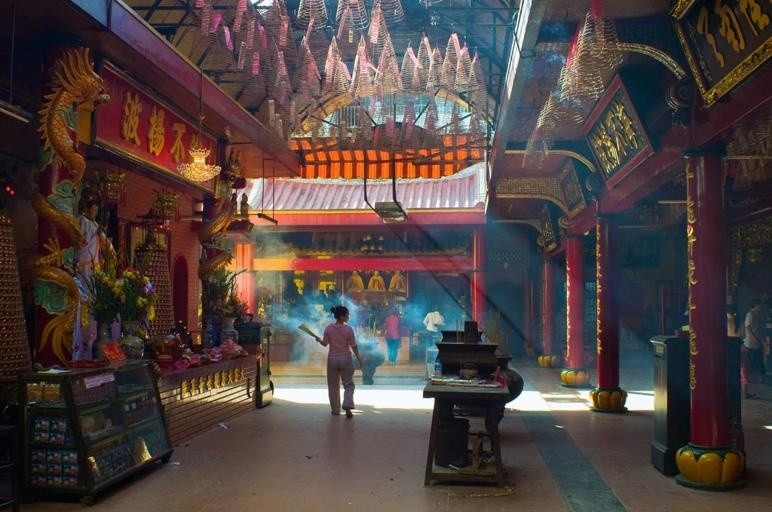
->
[423,373,509,490]
[15,358,175,506]
[649,334,747,476]
[237,322,273,408]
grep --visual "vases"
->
[220,316,239,346]
[200,316,219,348]
[117,321,145,360]
[91,320,114,360]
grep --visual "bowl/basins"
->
[436,339,498,370]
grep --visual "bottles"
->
[434,359,442,379]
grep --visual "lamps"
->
[178,69,223,184]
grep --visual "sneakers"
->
[330,411,339,415]
[345,408,354,417]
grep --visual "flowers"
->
[88,272,124,323]
[225,292,250,324]
[199,288,224,319]
[122,266,158,321]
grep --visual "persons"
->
[727,304,756,400]
[76,199,116,276]
[368,270,385,291]
[344,271,364,290]
[383,310,401,365]
[743,298,767,383]
[315,306,363,418]
[388,271,406,290]
[423,306,445,347]
